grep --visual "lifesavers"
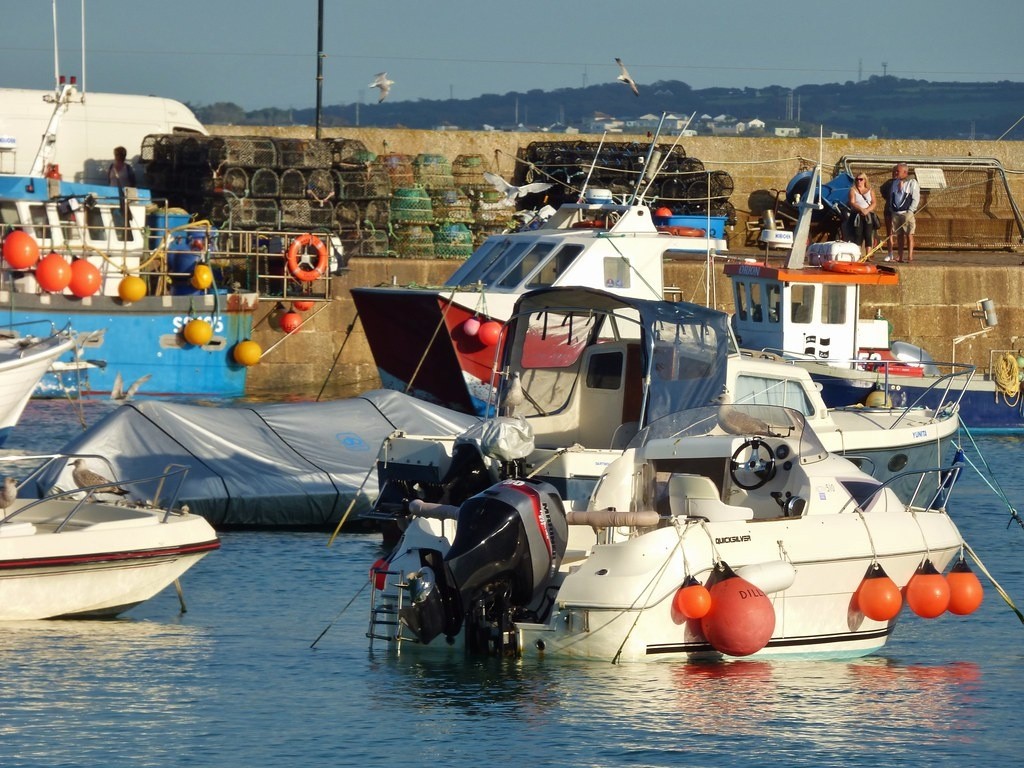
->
[287,233,329,281]
[657,225,707,237]
[822,260,876,275]
[570,218,614,228]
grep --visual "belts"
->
[895,211,907,214]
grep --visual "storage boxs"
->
[655,214,727,238]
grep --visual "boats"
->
[357,280,979,664]
[1,1,1023,525]
[0,453,223,625]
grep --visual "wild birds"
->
[369,71,394,104]
[0,477,19,522]
[615,57,640,97]
[67,459,132,505]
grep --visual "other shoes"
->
[907,259,914,264]
[896,256,902,262]
[883,254,893,262]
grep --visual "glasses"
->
[856,177,864,180]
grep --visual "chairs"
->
[669,475,754,522]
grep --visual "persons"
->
[849,172,876,262]
[306,174,335,203]
[108,147,135,187]
[880,162,920,263]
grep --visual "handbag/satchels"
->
[871,212,881,230]
